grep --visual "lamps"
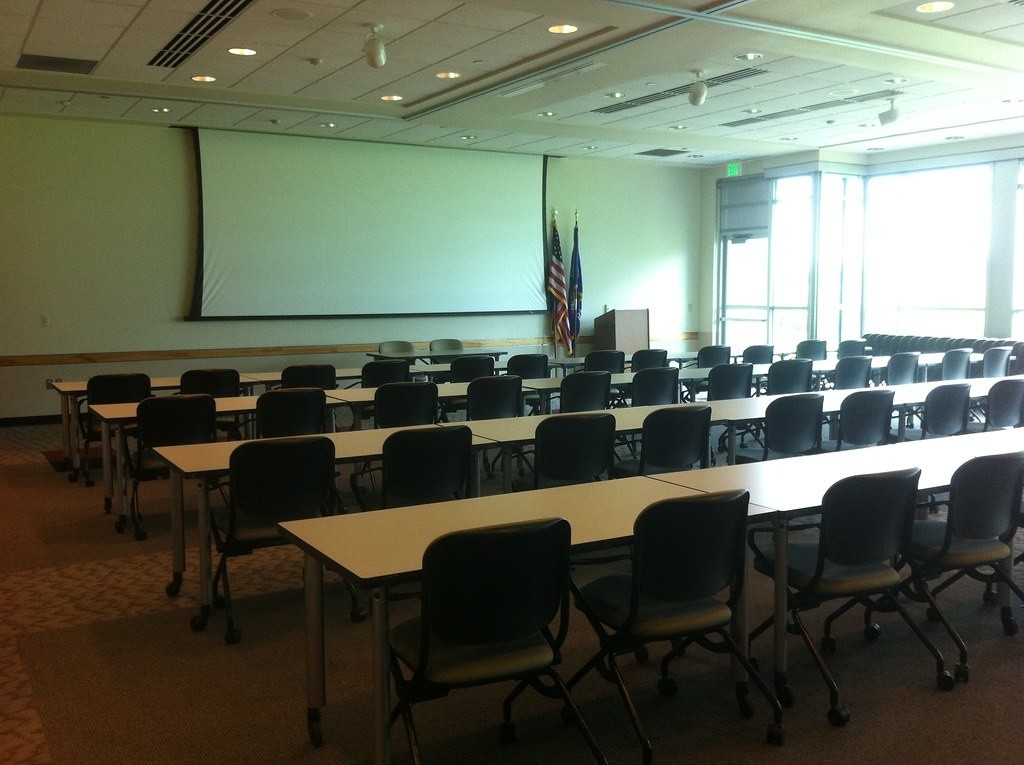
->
[879,97,900,126]
[361,24,388,69]
[689,68,708,106]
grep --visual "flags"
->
[548,220,574,356]
[568,225,582,356]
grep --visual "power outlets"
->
[47,378,53,390]
[55,378,63,390]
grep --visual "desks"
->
[52,375,259,483]
[522,372,691,415]
[700,387,905,465]
[883,374,1024,428]
[279,474,757,765]
[149,425,498,633]
[548,353,679,377]
[646,444,1019,710]
[366,348,508,376]
[437,401,728,494]
[896,427,1024,458]
[813,355,927,391]
[324,381,538,498]
[679,363,820,403]
[408,360,562,382]
[920,352,1016,382]
[240,367,418,396]
[87,394,348,534]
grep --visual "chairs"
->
[79,338,1024,765]
